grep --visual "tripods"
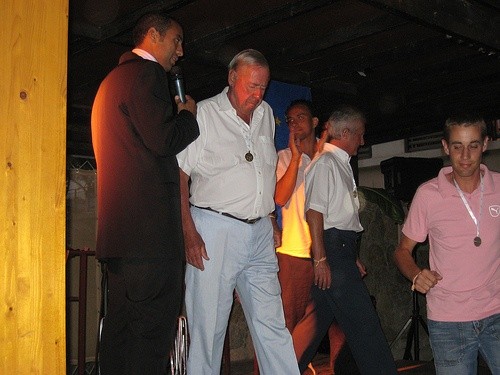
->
[389,291,429,362]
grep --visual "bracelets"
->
[411,271,421,291]
[314,256,326,266]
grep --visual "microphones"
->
[170,66,185,104]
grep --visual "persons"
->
[177,49,301,375]
[392,112,500,375]
[275,99,345,375]
[90,9,199,375]
[290,103,398,375]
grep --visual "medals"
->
[245,153,253,162]
[353,191,357,198]
[474,237,481,247]
